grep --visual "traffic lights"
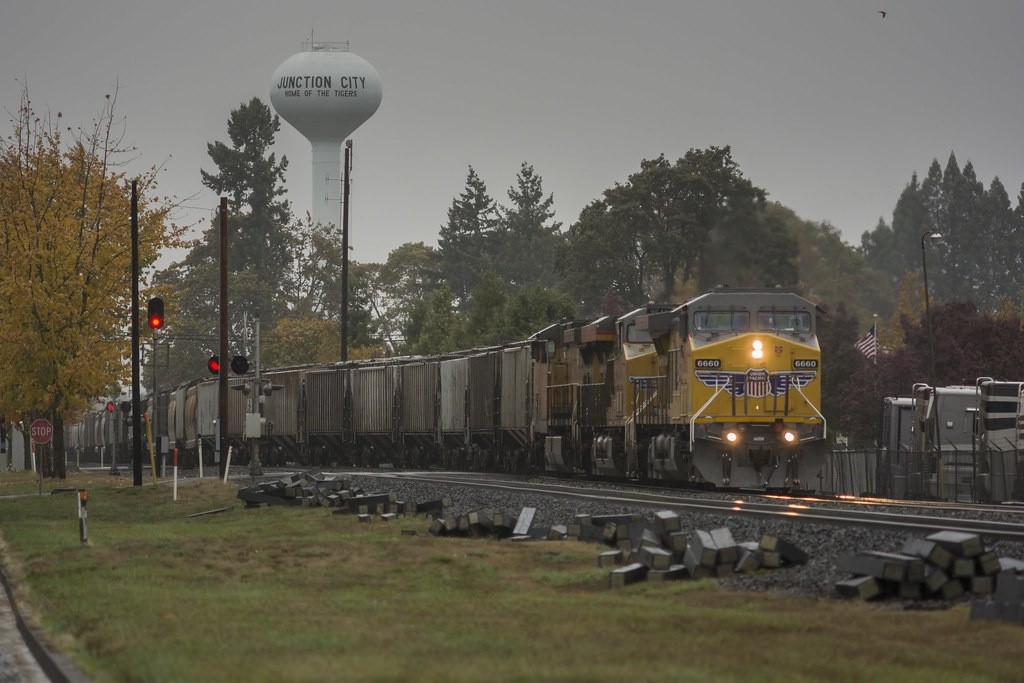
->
[146,296,164,331]
[106,400,114,413]
[207,356,221,374]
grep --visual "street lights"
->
[922,231,944,501]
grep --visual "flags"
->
[854,315,877,364]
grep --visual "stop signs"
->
[30,418,53,445]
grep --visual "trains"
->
[74,281,831,492]
